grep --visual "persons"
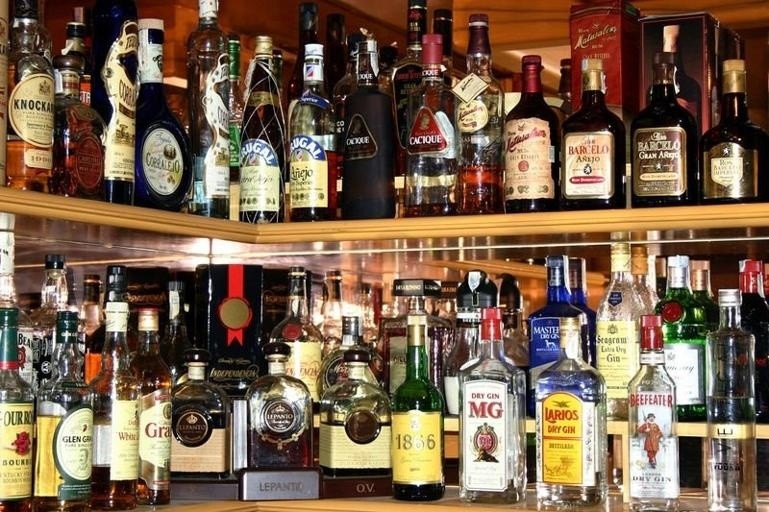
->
[639,413,666,465]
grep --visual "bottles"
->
[0,2,766,225]
[0,228,768,511]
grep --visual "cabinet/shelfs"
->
[0,187,769,511]
